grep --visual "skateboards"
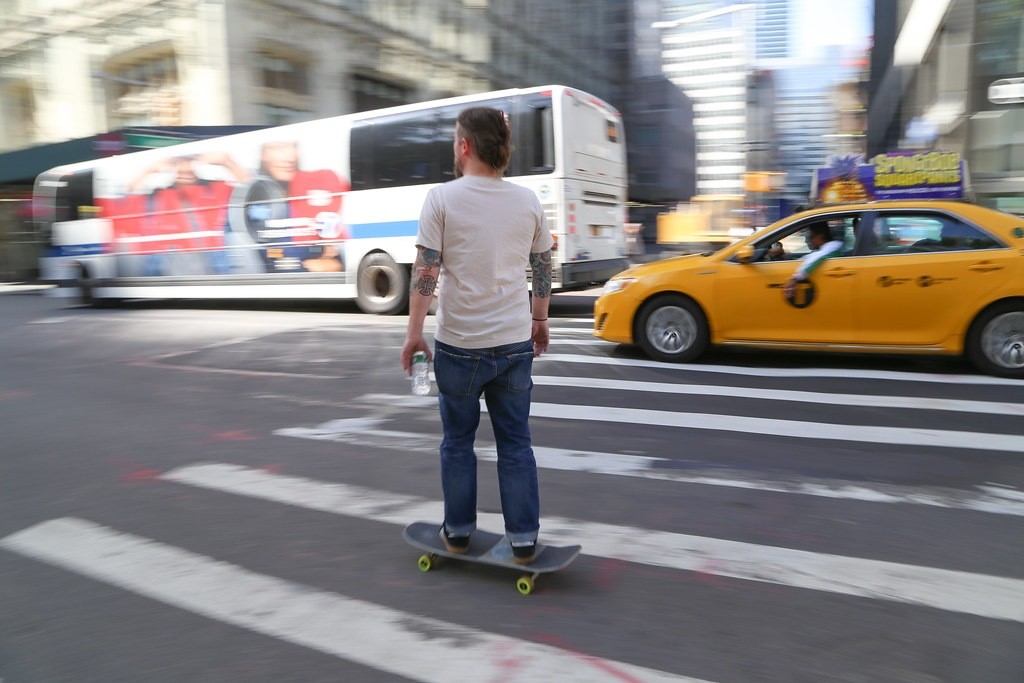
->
[401,520,583,596]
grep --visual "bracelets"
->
[532,318,547,321]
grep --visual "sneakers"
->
[438,525,470,554]
[510,539,537,564]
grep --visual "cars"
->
[591,150,1023,378]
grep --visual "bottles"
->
[411,351,430,396]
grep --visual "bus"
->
[30,83,632,314]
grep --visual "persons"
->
[766,221,844,281]
[125,141,352,273]
[402,106,554,564]
